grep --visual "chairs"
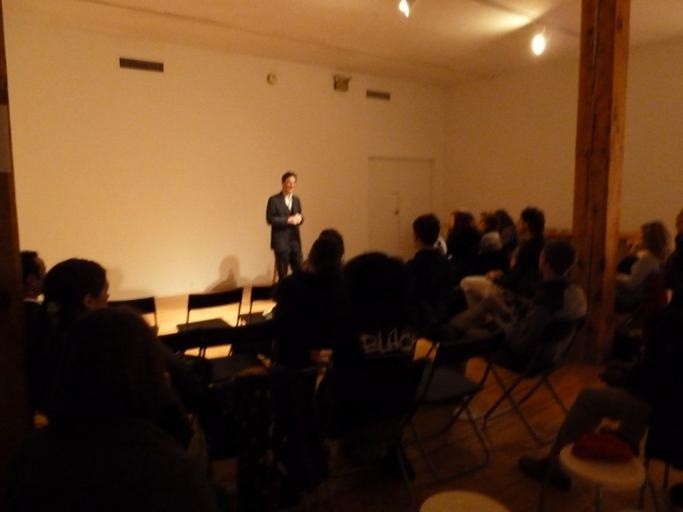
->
[397,321,582,481]
[109,285,279,364]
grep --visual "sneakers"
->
[518,457,572,491]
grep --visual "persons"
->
[20,248,236,511]
[266,171,305,275]
[260,203,682,480]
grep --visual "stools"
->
[420,439,646,511]
